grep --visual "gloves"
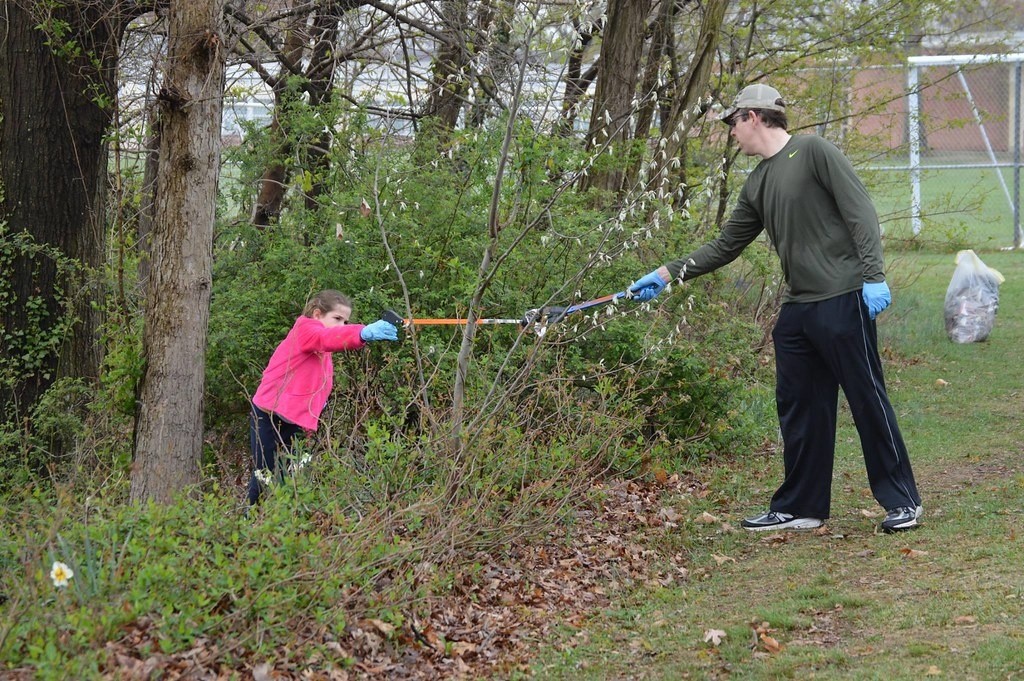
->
[625,269,667,303]
[360,320,398,342]
[861,280,892,320]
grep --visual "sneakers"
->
[741,511,822,532]
[881,505,924,528]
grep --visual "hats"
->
[719,83,785,125]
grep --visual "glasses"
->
[729,111,763,127]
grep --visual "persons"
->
[245,289,398,506]
[627,84,923,532]
